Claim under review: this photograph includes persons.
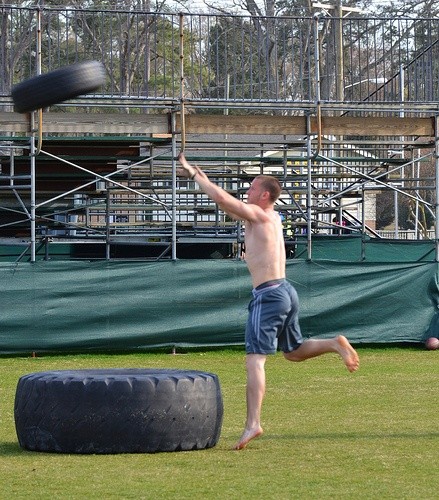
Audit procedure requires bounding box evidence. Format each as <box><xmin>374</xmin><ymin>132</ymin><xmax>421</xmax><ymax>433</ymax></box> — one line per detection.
<box><xmin>179</xmin><ymin>152</ymin><xmax>359</xmax><ymax>449</ymax></box>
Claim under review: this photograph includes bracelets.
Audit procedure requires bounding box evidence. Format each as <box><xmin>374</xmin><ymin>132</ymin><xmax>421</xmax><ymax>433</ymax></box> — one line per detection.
<box><xmin>191</xmin><ymin>171</ymin><xmax>198</xmax><ymax>179</ymax></box>
